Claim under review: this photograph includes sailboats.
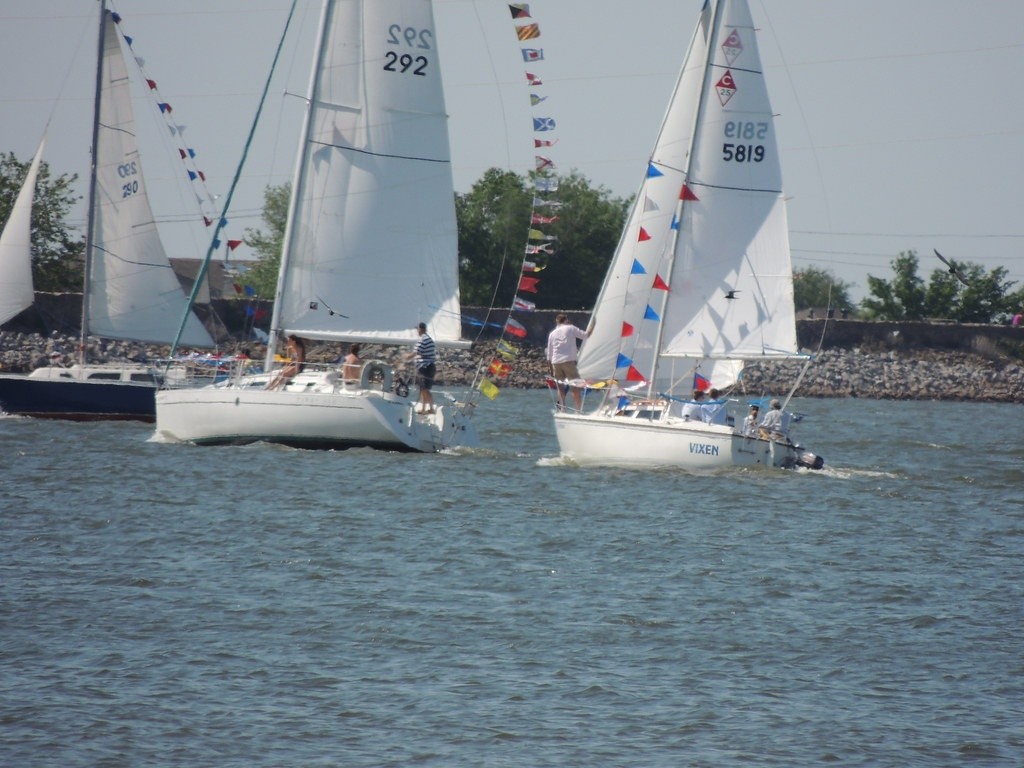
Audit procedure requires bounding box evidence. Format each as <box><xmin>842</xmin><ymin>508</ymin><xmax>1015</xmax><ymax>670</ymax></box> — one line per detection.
<box><xmin>147</xmin><ymin>0</ymin><xmax>557</xmax><ymax>453</ymax></box>
<box><xmin>550</xmin><ymin>0</ymin><xmax>822</xmax><ymax>472</ymax></box>
<box><xmin>1</xmin><ymin>0</ymin><xmax>271</xmax><ymax>419</ymax></box>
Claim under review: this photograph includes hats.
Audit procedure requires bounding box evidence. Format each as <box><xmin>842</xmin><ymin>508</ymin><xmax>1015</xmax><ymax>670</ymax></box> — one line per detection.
<box><xmin>693</xmin><ymin>389</ymin><xmax>704</xmax><ymax>396</ymax></box>
<box><xmin>750</xmin><ymin>404</ymin><xmax>759</xmax><ymax>410</ymax></box>
<box><xmin>769</xmin><ymin>400</ymin><xmax>782</xmax><ymax>408</ymax></box>
<box><xmin>414</xmin><ymin>321</ymin><xmax>427</xmax><ymax>329</ymax></box>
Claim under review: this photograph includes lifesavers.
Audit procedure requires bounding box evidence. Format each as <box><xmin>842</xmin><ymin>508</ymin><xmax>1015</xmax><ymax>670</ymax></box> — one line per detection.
<box><xmin>361</xmin><ymin>359</ymin><xmax>393</xmax><ymax>391</ymax></box>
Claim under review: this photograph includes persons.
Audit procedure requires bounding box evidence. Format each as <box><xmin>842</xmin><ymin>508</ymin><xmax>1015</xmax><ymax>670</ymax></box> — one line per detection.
<box><xmin>701</xmin><ymin>388</ymin><xmax>726</xmax><ymax>426</ymax></box>
<box><xmin>743</xmin><ymin>404</ymin><xmax>765</xmax><ymax>436</ymax></box>
<box><xmin>264</xmin><ymin>335</ymin><xmax>306</xmax><ymax>393</ymax></box>
<box><xmin>682</xmin><ymin>391</ymin><xmax>704</xmax><ymax>421</ymax></box>
<box><xmin>1013</xmin><ymin>312</ymin><xmax>1023</xmax><ymax>328</ymax></box>
<box><xmin>759</xmin><ymin>399</ymin><xmax>797</xmax><ymax>442</ymax></box>
<box><xmin>404</xmin><ymin>322</ymin><xmax>436</xmax><ymax>414</ymax></box>
<box><xmin>547</xmin><ymin>315</ymin><xmax>596</xmax><ymax>414</ymax></box>
<box><xmin>342</xmin><ymin>344</ymin><xmax>364</xmax><ymax>391</ymax></box>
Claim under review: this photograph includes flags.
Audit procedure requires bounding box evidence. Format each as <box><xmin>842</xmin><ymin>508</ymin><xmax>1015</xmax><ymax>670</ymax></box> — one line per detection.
<box><xmin>510</xmin><ymin>4</ymin><xmax>531</xmax><ymax>19</ymax></box>
<box><xmin>516</xmin><ymin>23</ymin><xmax>540</xmax><ymax>40</ymax></box>
<box><xmin>480</xmin><ymin>377</ymin><xmax>500</xmax><ymax>400</ymax></box>
<box><xmin>488</xmin><ymin>358</ymin><xmax>509</xmax><ymax>379</ymax></box>
<box><xmin>522</xmin><ymin>48</ymin><xmax>544</xmax><ymax>62</ymax></box>
<box><xmin>534</xmin><ymin>118</ymin><xmax>555</xmax><ymax>132</ymax></box>
<box><xmin>535</xmin><ymin>139</ymin><xmax>559</xmax><ymax>148</ymax></box>
<box><xmin>527</xmin><ymin>73</ymin><xmax>543</xmax><ymax>86</ymax></box>
<box><xmin>536</xmin><ymin>156</ymin><xmax>557</xmax><ymax>170</ymax></box>
<box><xmin>497</xmin><ymin>177</ymin><xmax>562</xmax><ymax>358</ymax></box>
<box><xmin>531</xmin><ymin>94</ymin><xmax>548</xmax><ymax>106</ymax></box>
